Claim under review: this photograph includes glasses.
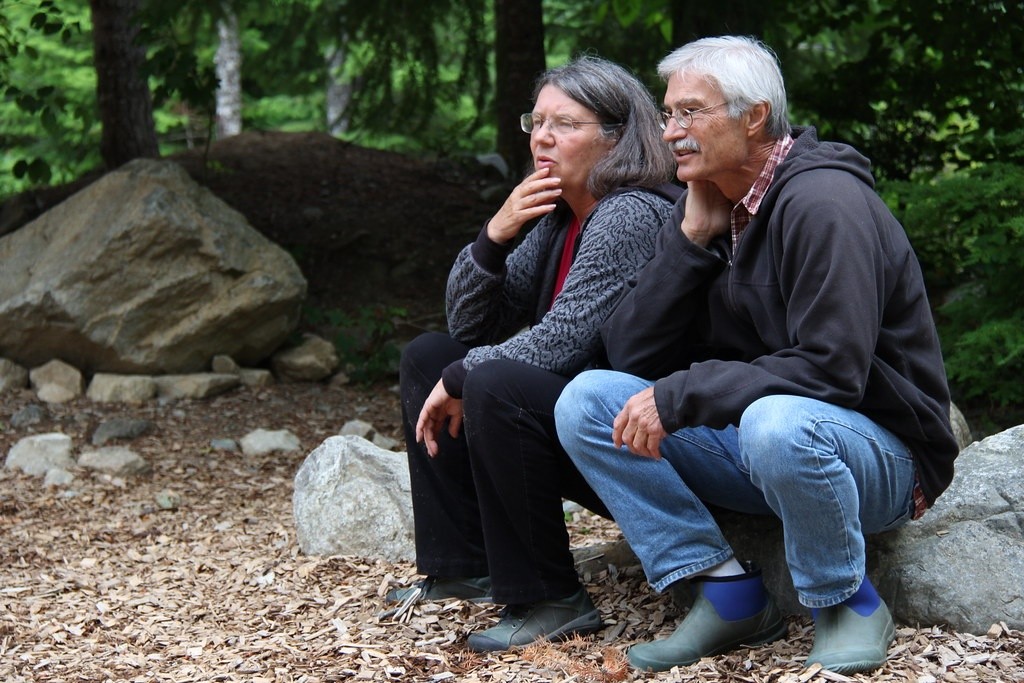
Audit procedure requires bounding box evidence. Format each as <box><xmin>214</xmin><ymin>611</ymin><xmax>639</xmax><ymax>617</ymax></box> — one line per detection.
<box><xmin>521</xmin><ymin>114</ymin><xmax>606</xmax><ymax>134</ymax></box>
<box><xmin>657</xmin><ymin>96</ymin><xmax>742</xmax><ymax>130</ymax></box>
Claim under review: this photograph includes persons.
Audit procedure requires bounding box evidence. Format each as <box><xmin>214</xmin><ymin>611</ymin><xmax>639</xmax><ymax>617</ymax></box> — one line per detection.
<box><xmin>552</xmin><ymin>34</ymin><xmax>960</xmax><ymax>671</ymax></box>
<box><xmin>379</xmin><ymin>57</ymin><xmax>690</xmax><ymax>651</ymax></box>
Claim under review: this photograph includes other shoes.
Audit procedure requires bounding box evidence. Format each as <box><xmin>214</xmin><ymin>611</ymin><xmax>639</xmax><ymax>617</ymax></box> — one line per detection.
<box><xmin>627</xmin><ymin>560</ymin><xmax>788</xmax><ymax>673</ymax></box>
<box><xmin>806</xmin><ymin>573</ymin><xmax>896</xmax><ymax>677</ymax></box>
<box><xmin>468</xmin><ymin>580</ymin><xmax>603</xmax><ymax>654</ymax></box>
<box><xmin>386</xmin><ymin>576</ymin><xmax>493</xmax><ymax>603</ymax></box>
